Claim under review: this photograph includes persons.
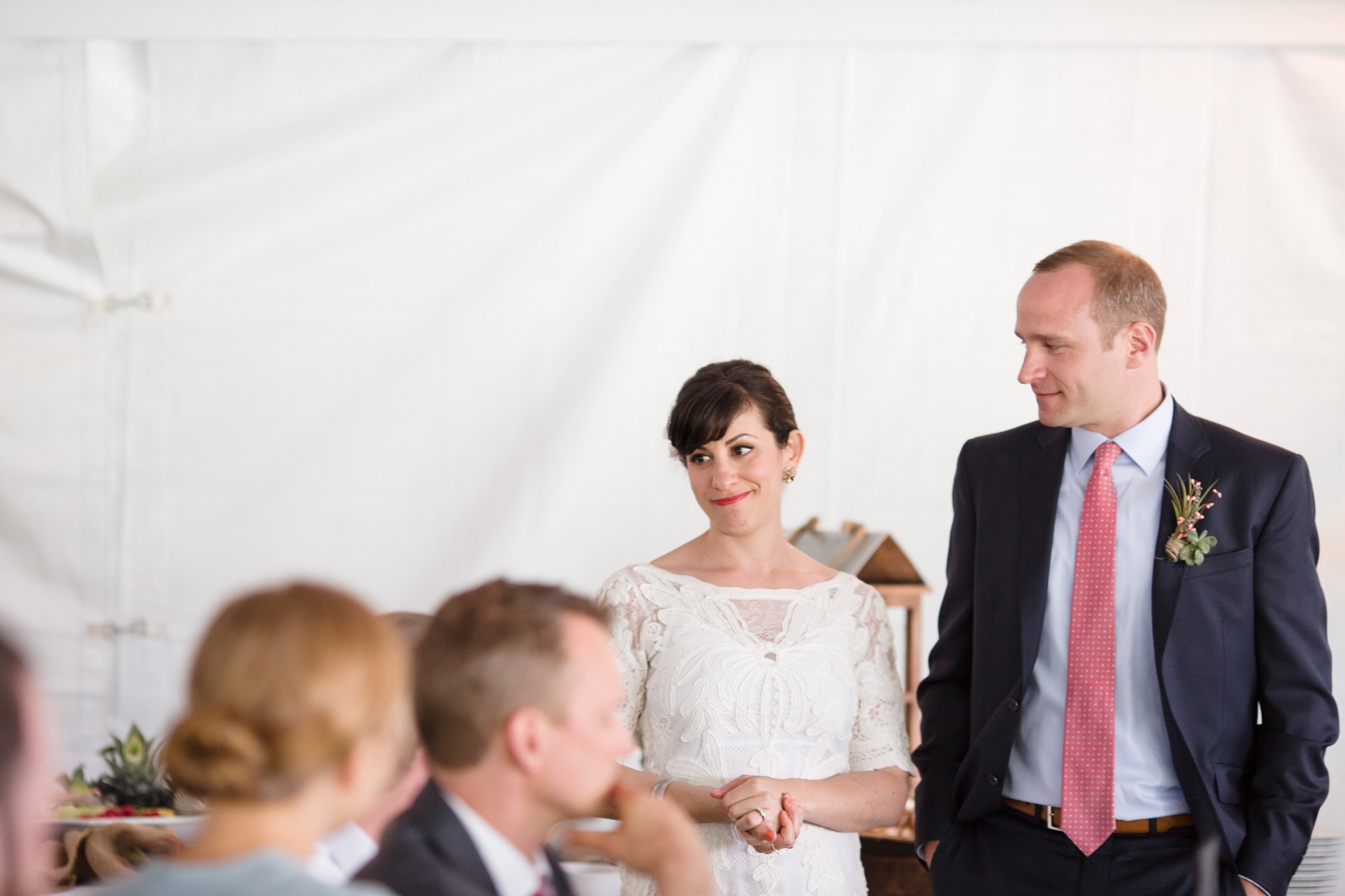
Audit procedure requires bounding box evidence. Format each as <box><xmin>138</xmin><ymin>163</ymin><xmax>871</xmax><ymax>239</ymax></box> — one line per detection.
<box><xmin>314</xmin><ymin>610</ymin><xmax>438</xmax><ymax>882</ymax></box>
<box><xmin>583</xmin><ymin>357</ymin><xmax>914</xmax><ymax>896</ymax></box>
<box><xmin>1</xmin><ymin>636</ymin><xmax>67</xmax><ymax>895</ymax></box>
<box><xmin>80</xmin><ymin>583</ymin><xmax>404</xmax><ymax>895</ymax></box>
<box><xmin>350</xmin><ymin>578</ymin><xmax>722</xmax><ymax>896</ymax></box>
<box><xmin>908</xmin><ymin>238</ymin><xmax>1344</xmax><ymax>896</ymax></box>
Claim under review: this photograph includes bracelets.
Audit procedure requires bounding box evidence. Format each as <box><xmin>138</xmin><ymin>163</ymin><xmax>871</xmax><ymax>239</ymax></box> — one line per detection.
<box><xmin>647</xmin><ymin>778</ymin><xmax>675</xmax><ymax>802</ymax></box>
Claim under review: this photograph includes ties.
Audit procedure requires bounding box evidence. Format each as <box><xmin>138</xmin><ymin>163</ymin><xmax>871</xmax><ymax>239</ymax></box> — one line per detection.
<box><xmin>1059</xmin><ymin>443</ymin><xmax>1127</xmax><ymax>857</ymax></box>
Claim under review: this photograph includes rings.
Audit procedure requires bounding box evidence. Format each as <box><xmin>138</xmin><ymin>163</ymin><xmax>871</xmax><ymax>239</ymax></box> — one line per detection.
<box><xmin>788</xmin><ymin>845</ymin><xmax>794</xmax><ymax>849</ymax></box>
<box><xmin>757</xmin><ymin>807</ymin><xmax>767</xmax><ymax>821</ymax></box>
<box><xmin>771</xmin><ymin>841</ymin><xmax>780</xmax><ymax>854</ymax></box>
<box><xmin>796</xmin><ymin>836</ymin><xmax>799</xmax><ymax>839</ymax></box>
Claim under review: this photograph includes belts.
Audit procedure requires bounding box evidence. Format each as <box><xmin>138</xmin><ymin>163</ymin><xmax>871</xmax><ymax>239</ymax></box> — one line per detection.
<box><xmin>998</xmin><ymin>797</ymin><xmax>1195</xmax><ymax>837</ymax></box>
